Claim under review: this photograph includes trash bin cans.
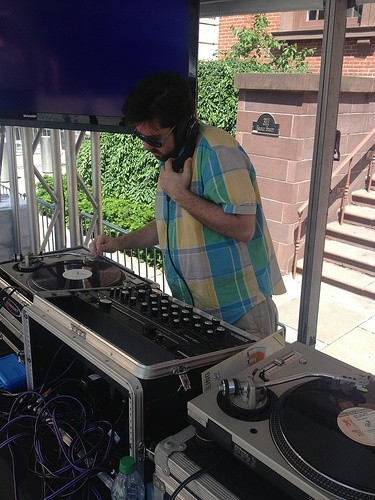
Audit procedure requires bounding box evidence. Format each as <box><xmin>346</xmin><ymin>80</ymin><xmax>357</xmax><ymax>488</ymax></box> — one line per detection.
<box><xmin>1</xmin><ymin>142</ymin><xmax>10</xmax><ymax>183</ymax></box>
<box><xmin>40</xmin><ymin>136</ymin><xmax>54</xmax><ymax>173</ymax></box>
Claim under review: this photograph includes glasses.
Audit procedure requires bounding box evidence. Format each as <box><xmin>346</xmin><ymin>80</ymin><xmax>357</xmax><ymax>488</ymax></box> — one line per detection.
<box><xmin>131</xmin><ymin>126</ymin><xmax>175</xmax><ymax>148</ymax></box>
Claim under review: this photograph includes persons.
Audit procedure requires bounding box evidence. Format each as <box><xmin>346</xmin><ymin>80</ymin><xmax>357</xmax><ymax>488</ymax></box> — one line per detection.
<box><xmin>86</xmin><ymin>68</ymin><xmax>290</xmax><ymax>341</ymax></box>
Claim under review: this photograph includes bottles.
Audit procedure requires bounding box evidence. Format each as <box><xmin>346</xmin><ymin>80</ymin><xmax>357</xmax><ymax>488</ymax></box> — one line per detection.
<box><xmin>111</xmin><ymin>456</ymin><xmax>146</xmax><ymax>500</ymax></box>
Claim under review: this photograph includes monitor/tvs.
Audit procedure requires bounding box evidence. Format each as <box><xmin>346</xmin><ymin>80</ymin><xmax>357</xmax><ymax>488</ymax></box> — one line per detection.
<box><xmin>0</xmin><ymin>0</ymin><xmax>201</xmax><ymax>129</ymax></box>
